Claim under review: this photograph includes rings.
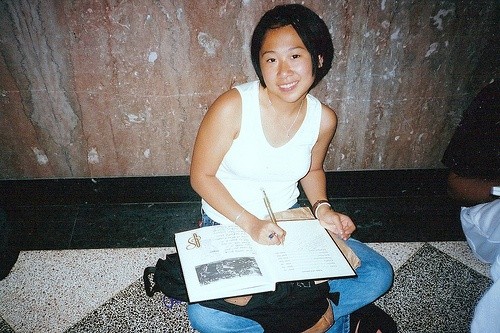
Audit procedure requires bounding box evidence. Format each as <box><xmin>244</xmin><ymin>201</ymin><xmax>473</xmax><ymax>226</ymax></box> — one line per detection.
<box><xmin>268</xmin><ymin>232</ymin><xmax>277</xmax><ymax>240</ymax></box>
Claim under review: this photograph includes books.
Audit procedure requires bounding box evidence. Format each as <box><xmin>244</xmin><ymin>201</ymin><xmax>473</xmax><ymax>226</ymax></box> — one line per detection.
<box><xmin>175</xmin><ymin>219</ymin><xmax>358</xmax><ymax>305</ymax></box>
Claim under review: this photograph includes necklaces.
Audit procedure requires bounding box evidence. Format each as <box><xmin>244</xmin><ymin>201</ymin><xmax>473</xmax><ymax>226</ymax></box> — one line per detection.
<box><xmin>266</xmin><ymin>88</ymin><xmax>303</xmax><ymax>138</ymax></box>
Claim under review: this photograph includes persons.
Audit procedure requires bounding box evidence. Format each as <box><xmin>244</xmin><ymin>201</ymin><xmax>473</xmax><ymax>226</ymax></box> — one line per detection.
<box><xmin>440</xmin><ymin>78</ymin><xmax>500</xmax><ymax>333</ymax></box>
<box><xmin>186</xmin><ymin>4</ymin><xmax>397</xmax><ymax>333</ymax></box>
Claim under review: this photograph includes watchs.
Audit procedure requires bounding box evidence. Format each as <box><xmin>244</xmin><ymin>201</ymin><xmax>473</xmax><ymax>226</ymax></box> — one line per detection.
<box><xmin>489</xmin><ymin>179</ymin><xmax>500</xmax><ymax>200</ymax></box>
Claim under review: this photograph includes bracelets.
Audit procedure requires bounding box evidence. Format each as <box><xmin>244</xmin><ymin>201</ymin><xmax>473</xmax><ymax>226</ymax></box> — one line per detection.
<box><xmin>234</xmin><ymin>209</ymin><xmax>246</xmax><ymax>224</ymax></box>
<box><xmin>311</xmin><ymin>200</ymin><xmax>333</xmax><ymax>219</ymax></box>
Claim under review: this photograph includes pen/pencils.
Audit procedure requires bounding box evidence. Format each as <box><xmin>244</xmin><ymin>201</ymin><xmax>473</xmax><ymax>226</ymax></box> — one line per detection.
<box><xmin>262</xmin><ymin>190</ymin><xmax>284</xmax><ymax>245</ymax></box>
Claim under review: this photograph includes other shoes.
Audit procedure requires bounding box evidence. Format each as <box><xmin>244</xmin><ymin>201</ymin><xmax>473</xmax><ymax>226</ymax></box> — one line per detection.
<box><xmin>350</xmin><ymin>302</ymin><xmax>397</xmax><ymax>333</ymax></box>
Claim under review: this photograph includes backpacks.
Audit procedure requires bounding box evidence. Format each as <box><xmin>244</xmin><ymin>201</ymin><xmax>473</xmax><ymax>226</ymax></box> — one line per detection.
<box><xmin>143</xmin><ymin>253</ymin><xmax>341</xmax><ymax>333</ymax></box>
